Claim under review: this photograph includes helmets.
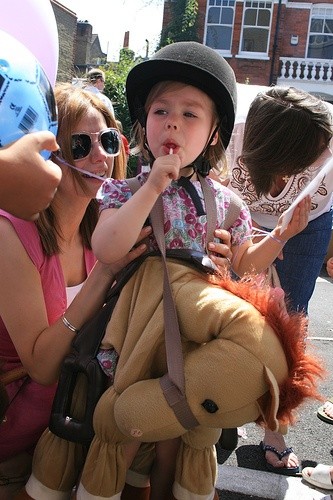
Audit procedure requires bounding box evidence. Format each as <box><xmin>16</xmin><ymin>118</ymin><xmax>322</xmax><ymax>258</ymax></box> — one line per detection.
<box><xmin>125</xmin><ymin>41</ymin><xmax>236</xmax><ymax>172</ymax></box>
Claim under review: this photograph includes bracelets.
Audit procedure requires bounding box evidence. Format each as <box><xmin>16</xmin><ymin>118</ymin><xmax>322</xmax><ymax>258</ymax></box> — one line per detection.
<box><xmin>92</xmin><ymin>42</ymin><xmax>312</xmax><ymax>500</ymax></box>
<box><xmin>61</xmin><ymin>313</ymin><xmax>80</xmax><ymax>334</ymax></box>
<box><xmin>269</xmin><ymin>233</ymin><xmax>287</xmax><ymax>247</ymax></box>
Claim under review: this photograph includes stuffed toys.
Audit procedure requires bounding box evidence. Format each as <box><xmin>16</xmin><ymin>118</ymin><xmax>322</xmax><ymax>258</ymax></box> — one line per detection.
<box><xmin>25</xmin><ymin>254</ymin><xmax>328</xmax><ymax>500</ymax></box>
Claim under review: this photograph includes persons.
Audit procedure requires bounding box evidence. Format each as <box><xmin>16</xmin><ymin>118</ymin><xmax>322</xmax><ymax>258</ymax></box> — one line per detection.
<box><xmin>302</xmin><ymin>256</ymin><xmax>333</xmax><ymax>491</ymax></box>
<box><xmin>0</xmin><ymin>130</ymin><xmax>63</xmax><ymax>220</ymax></box>
<box><xmin>209</xmin><ymin>87</ymin><xmax>333</xmax><ymax>475</ymax></box>
<box><xmin>0</xmin><ymin>83</ymin><xmax>231</xmax><ymax>500</ymax></box>
<box><xmin>83</xmin><ymin>69</ymin><xmax>115</xmax><ymax>120</ymax></box>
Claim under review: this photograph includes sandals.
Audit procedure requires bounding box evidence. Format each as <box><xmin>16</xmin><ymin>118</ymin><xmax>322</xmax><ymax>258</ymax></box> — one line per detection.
<box><xmin>317</xmin><ymin>397</ymin><xmax>333</xmax><ymax>425</ymax></box>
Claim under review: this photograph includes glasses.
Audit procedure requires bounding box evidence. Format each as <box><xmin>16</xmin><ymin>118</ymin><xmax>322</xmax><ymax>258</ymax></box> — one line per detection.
<box><xmin>68</xmin><ymin>127</ymin><xmax>122</xmax><ymax>161</ymax></box>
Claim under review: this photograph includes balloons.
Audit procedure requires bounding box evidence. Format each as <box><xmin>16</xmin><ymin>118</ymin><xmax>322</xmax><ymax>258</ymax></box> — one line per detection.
<box><xmin>0</xmin><ymin>0</ymin><xmax>60</xmax><ymax>88</ymax></box>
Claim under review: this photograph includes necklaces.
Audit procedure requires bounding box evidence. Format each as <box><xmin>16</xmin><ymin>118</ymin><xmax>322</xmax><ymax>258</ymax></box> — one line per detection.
<box><xmin>282</xmin><ymin>175</ymin><xmax>290</xmax><ymax>184</ymax></box>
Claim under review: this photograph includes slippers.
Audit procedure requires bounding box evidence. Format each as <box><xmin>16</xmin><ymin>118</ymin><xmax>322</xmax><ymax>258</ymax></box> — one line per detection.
<box><xmin>260</xmin><ymin>441</ymin><xmax>300</xmax><ymax>473</ymax></box>
<box><xmin>302</xmin><ymin>464</ymin><xmax>333</xmax><ymax>489</ymax></box>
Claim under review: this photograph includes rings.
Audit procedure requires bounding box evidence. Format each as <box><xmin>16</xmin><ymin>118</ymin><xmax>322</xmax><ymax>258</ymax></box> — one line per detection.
<box><xmin>226</xmin><ymin>257</ymin><xmax>230</xmax><ymax>264</ymax></box>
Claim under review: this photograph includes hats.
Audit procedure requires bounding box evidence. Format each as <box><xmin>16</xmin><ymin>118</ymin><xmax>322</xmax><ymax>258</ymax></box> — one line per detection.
<box><xmin>87</xmin><ymin>69</ymin><xmax>106</xmax><ymax>80</ymax></box>
